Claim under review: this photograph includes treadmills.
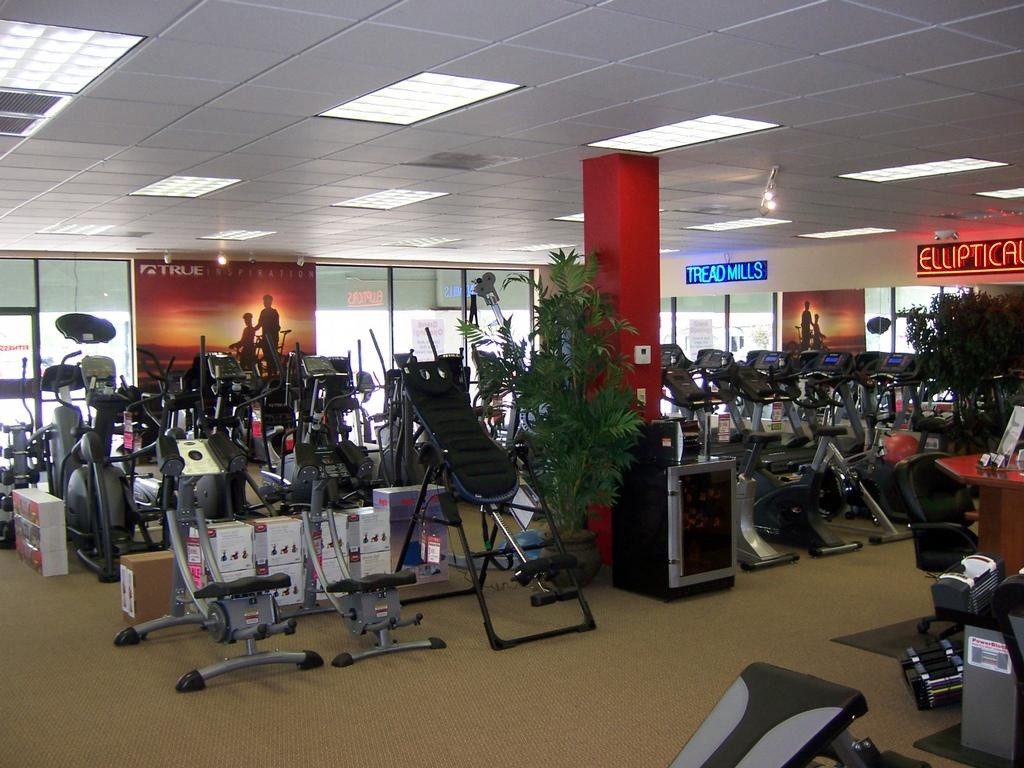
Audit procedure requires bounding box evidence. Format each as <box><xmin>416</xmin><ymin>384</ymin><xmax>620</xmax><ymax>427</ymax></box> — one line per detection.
<box><xmin>660</xmin><ymin>343</ymin><xmax>926</xmax><ymax>474</ymax></box>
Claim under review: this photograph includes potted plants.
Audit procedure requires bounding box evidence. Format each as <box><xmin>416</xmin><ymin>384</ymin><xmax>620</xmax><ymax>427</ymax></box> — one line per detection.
<box><xmin>455</xmin><ymin>245</ymin><xmax>649</xmax><ymax>588</ymax></box>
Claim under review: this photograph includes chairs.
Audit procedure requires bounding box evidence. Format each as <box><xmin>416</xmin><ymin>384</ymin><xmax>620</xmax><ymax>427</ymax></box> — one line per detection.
<box><xmin>892</xmin><ymin>450</ymin><xmax>981</xmax><ymax>573</ymax></box>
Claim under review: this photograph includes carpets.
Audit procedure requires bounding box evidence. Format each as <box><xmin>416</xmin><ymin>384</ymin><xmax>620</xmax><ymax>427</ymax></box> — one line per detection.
<box><xmin>913</xmin><ymin>723</ymin><xmax>1024</xmax><ymax>768</ymax></box>
<box><xmin>829</xmin><ymin>614</ymin><xmax>964</xmax><ymax>659</ymax></box>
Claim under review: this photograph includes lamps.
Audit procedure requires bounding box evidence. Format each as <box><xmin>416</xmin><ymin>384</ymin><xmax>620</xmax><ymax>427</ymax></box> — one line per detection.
<box><xmin>934</xmin><ymin>231</ymin><xmax>960</xmax><ymax>241</ymax></box>
<box><xmin>760</xmin><ymin>166</ymin><xmax>781</xmax><ymax>215</ymax></box>
<box><xmin>248</xmin><ymin>252</ymin><xmax>256</xmax><ymax>264</ymax></box>
<box><xmin>164</xmin><ymin>249</ymin><xmax>172</xmax><ymax>264</ymax></box>
<box><xmin>296</xmin><ymin>255</ymin><xmax>305</xmax><ymax>266</ymax></box>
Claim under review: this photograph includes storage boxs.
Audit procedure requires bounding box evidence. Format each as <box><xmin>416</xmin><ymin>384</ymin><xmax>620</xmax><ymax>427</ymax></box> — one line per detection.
<box><xmin>120</xmin><ymin>551</ymin><xmax>176</xmax><ymax>626</ymax></box>
<box><xmin>187</xmin><ymin>484</ymin><xmax>450</xmax><ymax>613</ymax></box>
<box><xmin>12</xmin><ymin>488</ymin><xmax>68</xmax><ymax>576</ymax></box>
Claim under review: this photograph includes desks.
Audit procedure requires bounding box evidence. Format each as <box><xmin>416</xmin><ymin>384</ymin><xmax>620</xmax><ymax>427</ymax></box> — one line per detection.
<box><xmin>935</xmin><ymin>453</ymin><xmax>1024</xmax><ymax>579</ymax></box>
